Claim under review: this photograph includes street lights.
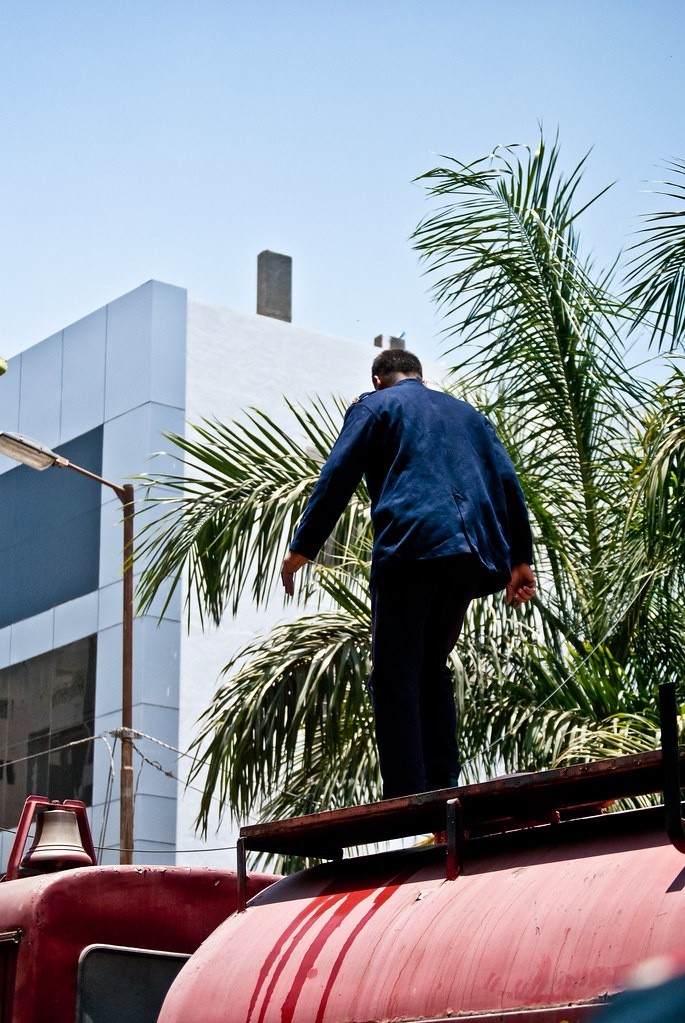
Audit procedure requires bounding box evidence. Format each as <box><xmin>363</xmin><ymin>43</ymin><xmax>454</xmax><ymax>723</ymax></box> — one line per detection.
<box><xmin>0</xmin><ymin>430</ymin><xmax>134</xmax><ymax>865</ymax></box>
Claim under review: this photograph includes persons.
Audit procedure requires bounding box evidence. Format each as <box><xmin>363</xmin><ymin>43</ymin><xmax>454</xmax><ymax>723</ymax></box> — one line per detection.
<box><xmin>278</xmin><ymin>349</ymin><xmax>538</xmax><ymax>803</ymax></box>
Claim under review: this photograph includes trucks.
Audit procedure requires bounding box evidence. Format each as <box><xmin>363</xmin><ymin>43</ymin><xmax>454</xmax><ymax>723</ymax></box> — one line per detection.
<box><xmin>0</xmin><ymin>683</ymin><xmax>685</xmax><ymax>1023</ymax></box>
